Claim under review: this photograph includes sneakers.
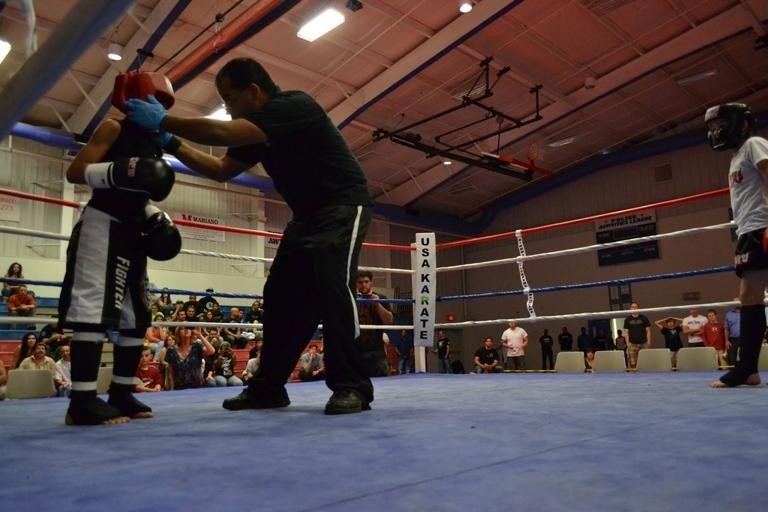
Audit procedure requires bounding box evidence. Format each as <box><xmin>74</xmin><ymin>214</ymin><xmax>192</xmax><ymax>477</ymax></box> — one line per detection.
<box><xmin>222</xmin><ymin>383</ymin><xmax>290</xmax><ymax>410</ymax></box>
<box><xmin>325</xmin><ymin>390</ymin><xmax>363</xmax><ymax>413</ymax></box>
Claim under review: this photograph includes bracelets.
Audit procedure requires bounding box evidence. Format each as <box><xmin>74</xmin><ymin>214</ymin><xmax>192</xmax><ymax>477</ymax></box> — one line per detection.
<box><xmin>162</xmin><ymin>136</ymin><xmax>180</xmax><ymax>155</ymax></box>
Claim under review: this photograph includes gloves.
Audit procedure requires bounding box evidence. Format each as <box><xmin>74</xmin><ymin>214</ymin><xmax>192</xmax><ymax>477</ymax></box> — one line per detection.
<box><xmin>126</xmin><ymin>94</ymin><xmax>173</xmax><ymax>147</ymax></box>
<box><xmin>113</xmin><ymin>158</ymin><xmax>174</xmax><ymax>201</ymax></box>
<box><xmin>144</xmin><ymin>211</ymin><xmax>181</xmax><ymax>260</ymax></box>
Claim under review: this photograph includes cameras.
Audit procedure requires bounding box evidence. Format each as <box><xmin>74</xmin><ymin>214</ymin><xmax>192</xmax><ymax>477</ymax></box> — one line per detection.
<box><xmin>186</xmin><ymin>326</ymin><xmax>195</xmax><ymax>330</ymax></box>
<box><xmin>357</xmin><ymin>294</ymin><xmax>372</xmax><ymax>300</ymax></box>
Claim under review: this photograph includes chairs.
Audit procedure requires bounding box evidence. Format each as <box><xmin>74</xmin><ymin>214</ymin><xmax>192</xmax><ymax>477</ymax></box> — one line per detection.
<box><xmin>552</xmin><ymin>342</ymin><xmax>767</xmax><ymax>378</ymax></box>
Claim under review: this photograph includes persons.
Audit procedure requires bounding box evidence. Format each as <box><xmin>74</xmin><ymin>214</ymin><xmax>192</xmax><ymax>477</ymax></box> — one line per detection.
<box><xmin>431</xmin><ymin>329</ymin><xmax>450</xmax><ymax>375</ymax></box>
<box><xmin>57</xmin><ymin>70</ymin><xmax>181</xmax><ymax>426</ymax></box>
<box><xmin>473</xmin><ymin>289</ymin><xmax>767</xmax><ymax>374</ymax></box>
<box><xmin>706</xmin><ymin>103</ymin><xmax>768</xmax><ymax>387</ymax></box>
<box><xmin>122</xmin><ymin>57</ymin><xmax>377</xmax><ymax>415</ymax></box>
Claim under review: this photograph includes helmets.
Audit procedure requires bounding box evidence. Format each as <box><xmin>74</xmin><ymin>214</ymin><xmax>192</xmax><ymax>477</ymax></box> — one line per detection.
<box><xmin>111</xmin><ymin>72</ymin><xmax>175</xmax><ymax>114</ymax></box>
<box><xmin>705</xmin><ymin>104</ymin><xmax>754</xmax><ymax>152</ymax></box>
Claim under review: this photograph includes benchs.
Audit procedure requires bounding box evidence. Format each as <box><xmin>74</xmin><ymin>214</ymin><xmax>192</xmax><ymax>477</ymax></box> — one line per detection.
<box><xmin>0</xmin><ymin>295</ymin><xmax>324</xmax><ymax>403</ymax></box>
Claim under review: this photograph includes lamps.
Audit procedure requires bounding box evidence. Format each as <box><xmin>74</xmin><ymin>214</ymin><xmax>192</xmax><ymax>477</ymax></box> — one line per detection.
<box><xmin>105</xmin><ymin>43</ymin><xmax>124</xmax><ymax>61</ymax></box>
